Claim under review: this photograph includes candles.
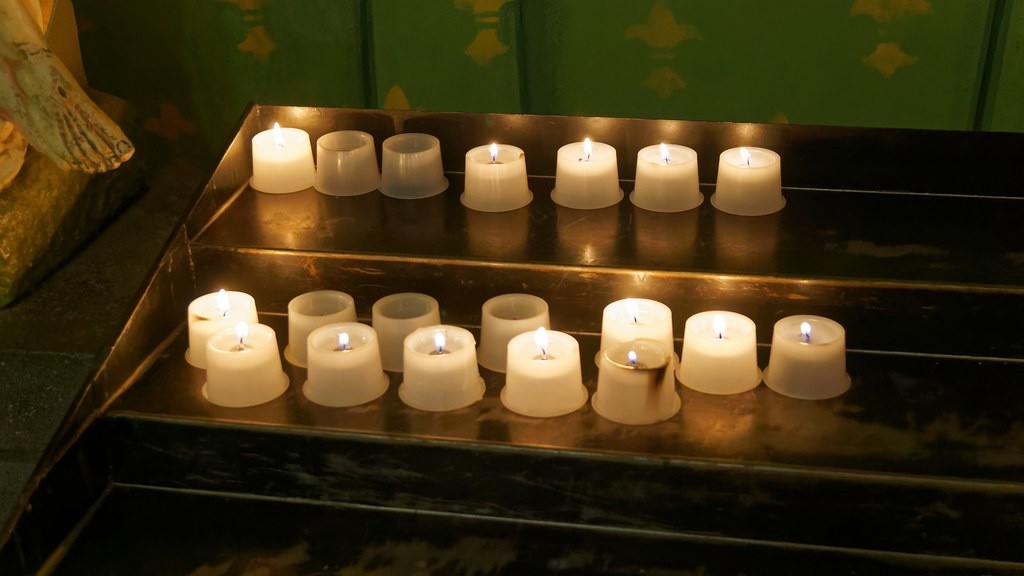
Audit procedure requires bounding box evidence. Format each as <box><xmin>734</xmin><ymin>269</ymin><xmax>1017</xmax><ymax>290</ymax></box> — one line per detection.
<box><xmin>372</xmin><ymin>293</ymin><xmax>440</xmax><ymax>373</ymax></box>
<box><xmin>710</xmin><ymin>146</ymin><xmax>787</xmax><ymax>216</ymax></box>
<box><xmin>762</xmin><ymin>313</ymin><xmax>852</xmax><ymax>401</ymax></box>
<box><xmin>398</xmin><ymin>324</ymin><xmax>486</xmax><ymax>412</ymax></box>
<box><xmin>591</xmin><ymin>336</ymin><xmax>681</xmax><ymax>425</ymax></box>
<box><xmin>283</xmin><ymin>290</ymin><xmax>356</xmax><ymax>368</ymax></box>
<box><xmin>201</xmin><ymin>319</ymin><xmax>290</xmax><ymax>408</ymax></box>
<box><xmin>594</xmin><ymin>297</ymin><xmax>679</xmax><ymax>372</ymax></box>
<box><xmin>500</xmin><ymin>328</ymin><xmax>589</xmax><ymax>418</ymax></box>
<box><xmin>459</xmin><ymin>141</ymin><xmax>534</xmax><ymax>213</ymax></box>
<box><xmin>185</xmin><ymin>288</ymin><xmax>259</xmax><ymax>370</ymax></box>
<box><xmin>675</xmin><ymin>311</ymin><xmax>763</xmax><ymax>395</ymax></box>
<box><xmin>313</xmin><ymin>130</ymin><xmax>381</xmax><ymax>196</ymax></box>
<box><xmin>550</xmin><ymin>137</ymin><xmax>624</xmax><ymax>210</ymax></box>
<box><xmin>302</xmin><ymin>321</ymin><xmax>391</xmax><ymax>407</ymax></box>
<box><xmin>249</xmin><ymin>120</ymin><xmax>315</xmax><ymax>194</ymax></box>
<box><xmin>377</xmin><ymin>133</ymin><xmax>449</xmax><ymax>199</ymax></box>
<box><xmin>629</xmin><ymin>141</ymin><xmax>704</xmax><ymax>212</ymax></box>
<box><xmin>476</xmin><ymin>293</ymin><xmax>551</xmax><ymax>373</ymax></box>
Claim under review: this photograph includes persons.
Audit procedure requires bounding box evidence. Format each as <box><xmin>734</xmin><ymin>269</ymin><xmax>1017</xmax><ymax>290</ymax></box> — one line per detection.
<box><xmin>2</xmin><ymin>1</ymin><xmax>135</xmax><ymax>174</ymax></box>
